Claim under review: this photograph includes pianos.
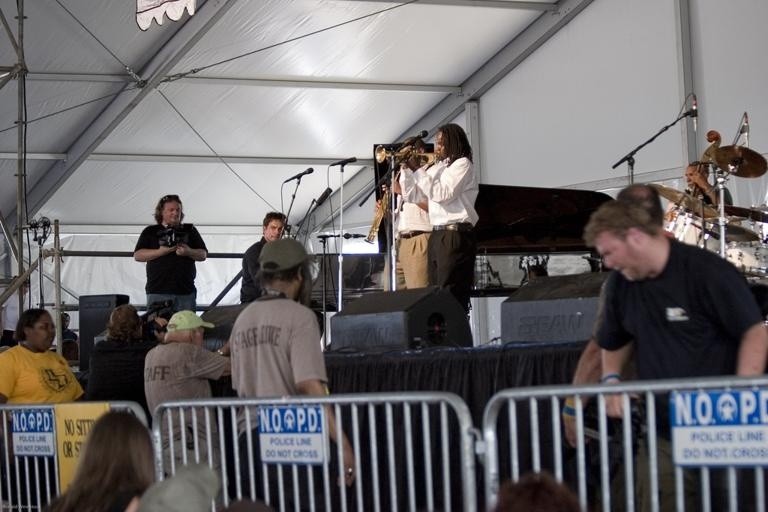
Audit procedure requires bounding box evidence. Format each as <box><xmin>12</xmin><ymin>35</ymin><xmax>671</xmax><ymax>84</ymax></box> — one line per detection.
<box><xmin>475</xmin><ymin>183</ymin><xmax>618</xmax><ymax>274</ymax></box>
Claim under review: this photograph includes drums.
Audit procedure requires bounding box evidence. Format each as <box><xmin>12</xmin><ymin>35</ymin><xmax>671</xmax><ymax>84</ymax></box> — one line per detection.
<box><xmin>720</xmin><ymin>240</ymin><xmax>767</xmax><ymax>284</ymax></box>
<box><xmin>669</xmin><ymin>213</ymin><xmax>720</xmax><ymax>255</ymax></box>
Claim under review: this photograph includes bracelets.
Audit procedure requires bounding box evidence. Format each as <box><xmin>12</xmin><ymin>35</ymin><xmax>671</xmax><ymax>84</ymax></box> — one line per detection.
<box><xmin>703</xmin><ymin>183</ymin><xmax>712</xmax><ymax>192</ymax></box>
<box><xmin>218</xmin><ymin>349</ymin><xmax>224</xmax><ymax>355</ymax></box>
<box><xmin>601</xmin><ymin>372</ymin><xmax>621</xmax><ymax>384</ymax></box>
<box><xmin>563</xmin><ymin>395</ymin><xmax>576</xmax><ymax>420</ymax></box>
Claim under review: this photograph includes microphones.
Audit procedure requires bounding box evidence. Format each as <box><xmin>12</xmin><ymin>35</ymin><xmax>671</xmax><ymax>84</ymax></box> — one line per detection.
<box><xmin>691</xmin><ymin>98</ymin><xmax>699</xmax><ymax>134</ymax></box>
<box><xmin>314</xmin><ymin>186</ymin><xmax>333</xmax><ymax>206</ymax></box>
<box><xmin>744</xmin><ymin>114</ymin><xmax>750</xmax><ymax>148</ymax></box>
<box><xmin>284</xmin><ymin>166</ymin><xmax>314</xmax><ymax>181</ymax></box>
<box><xmin>405</xmin><ymin>129</ymin><xmax>428</xmax><ymax>146</ymax></box>
<box><xmin>331</xmin><ymin>155</ymin><xmax>358</xmax><ymax>168</ymax></box>
<box><xmin>352</xmin><ymin>232</ymin><xmax>367</xmax><ymax>239</ymax></box>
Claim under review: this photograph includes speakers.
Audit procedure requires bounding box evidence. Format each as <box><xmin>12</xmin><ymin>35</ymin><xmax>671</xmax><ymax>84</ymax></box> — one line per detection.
<box><xmin>331</xmin><ymin>286</ymin><xmax>474</xmax><ymax>351</ymax></box>
<box><xmin>199</xmin><ymin>304</ymin><xmax>249</xmax><ymax>340</ymax></box>
<box><xmin>500</xmin><ymin>270</ymin><xmax>614</xmax><ymax>343</ymax></box>
<box><xmin>79</xmin><ymin>294</ymin><xmax>131</xmax><ymax>370</ymax></box>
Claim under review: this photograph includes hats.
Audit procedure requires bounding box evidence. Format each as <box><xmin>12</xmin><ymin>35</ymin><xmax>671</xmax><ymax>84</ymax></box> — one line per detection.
<box><xmin>259</xmin><ymin>238</ymin><xmax>309</xmax><ymax>273</ymax></box>
<box><xmin>165</xmin><ymin>309</ymin><xmax>215</xmax><ymax>333</ymax></box>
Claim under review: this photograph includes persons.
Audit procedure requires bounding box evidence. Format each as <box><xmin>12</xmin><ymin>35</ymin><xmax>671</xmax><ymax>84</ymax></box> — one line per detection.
<box><xmin>134</xmin><ymin>195</ymin><xmax>208</xmax><ymax>313</ymax></box>
<box><xmin>0</xmin><ymin>278</ymin><xmax>36</xmax><ymax>347</ymax></box>
<box><xmin>0</xmin><ymin>308</ymin><xmax>84</xmax><ymax>512</ymax></box>
<box><xmin>584</xmin><ymin>200</ymin><xmax>768</xmax><ymax>511</ymax></box>
<box><xmin>374</xmin><ymin>136</ymin><xmax>433</xmax><ymax>293</ymax></box>
<box><xmin>561</xmin><ymin>182</ymin><xmax>674</xmax><ymax>512</ymax></box>
<box><xmin>61</xmin><ymin>313</ymin><xmax>77</xmax><ymax>341</ymax></box>
<box><xmin>240</xmin><ymin>212</ymin><xmax>287</xmax><ymax>303</ymax></box>
<box><xmin>666</xmin><ymin>160</ymin><xmax>734</xmax><ymax>219</ymax></box>
<box><xmin>229</xmin><ymin>238</ymin><xmax>359</xmax><ymax>511</ymax></box>
<box><xmin>145</xmin><ymin>310</ymin><xmax>231</xmax><ymax>512</ymax></box>
<box><xmin>493</xmin><ymin>470</ymin><xmax>579</xmax><ymax>510</ymax></box>
<box><xmin>399</xmin><ymin>125</ymin><xmax>479</xmax><ymax>312</ymax></box>
<box><xmin>86</xmin><ymin>305</ymin><xmax>167</xmax><ymax>429</ymax></box>
<box><xmin>41</xmin><ymin>410</ymin><xmax>156</xmax><ymax>512</ymax></box>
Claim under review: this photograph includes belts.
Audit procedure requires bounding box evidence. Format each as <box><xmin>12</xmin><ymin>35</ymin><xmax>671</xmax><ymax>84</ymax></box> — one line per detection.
<box><xmin>399</xmin><ymin>230</ymin><xmax>423</xmax><ymax>239</ymax></box>
<box><xmin>433</xmin><ymin>224</ymin><xmax>470</xmax><ymax>233</ymax></box>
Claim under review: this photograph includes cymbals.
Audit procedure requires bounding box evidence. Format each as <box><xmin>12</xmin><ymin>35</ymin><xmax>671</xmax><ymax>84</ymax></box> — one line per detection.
<box><xmin>711</xmin><ymin>147</ymin><xmax>766</xmax><ymax>177</ymax></box>
<box><xmin>706</xmin><ymin>224</ymin><xmax>760</xmax><ymax>243</ymax></box>
<box><xmin>650</xmin><ymin>183</ymin><xmax>720</xmax><ymax>216</ymax></box>
<box><xmin>709</xmin><ymin>206</ymin><xmax>767</xmax><ymax>222</ymax></box>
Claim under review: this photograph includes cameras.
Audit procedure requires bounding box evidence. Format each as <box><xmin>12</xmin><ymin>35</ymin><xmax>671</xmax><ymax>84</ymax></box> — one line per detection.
<box><xmin>161</xmin><ymin>223</ymin><xmax>186</xmax><ymax>246</ymax></box>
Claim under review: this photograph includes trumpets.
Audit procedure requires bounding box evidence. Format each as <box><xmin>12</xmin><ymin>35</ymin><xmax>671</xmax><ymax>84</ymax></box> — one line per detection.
<box><xmin>375</xmin><ymin>145</ymin><xmax>435</xmax><ymax>162</ymax></box>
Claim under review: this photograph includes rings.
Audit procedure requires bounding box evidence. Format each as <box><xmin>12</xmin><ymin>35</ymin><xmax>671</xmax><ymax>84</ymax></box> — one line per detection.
<box><xmin>349</xmin><ymin>468</ymin><xmax>353</xmax><ymax>476</ymax></box>
